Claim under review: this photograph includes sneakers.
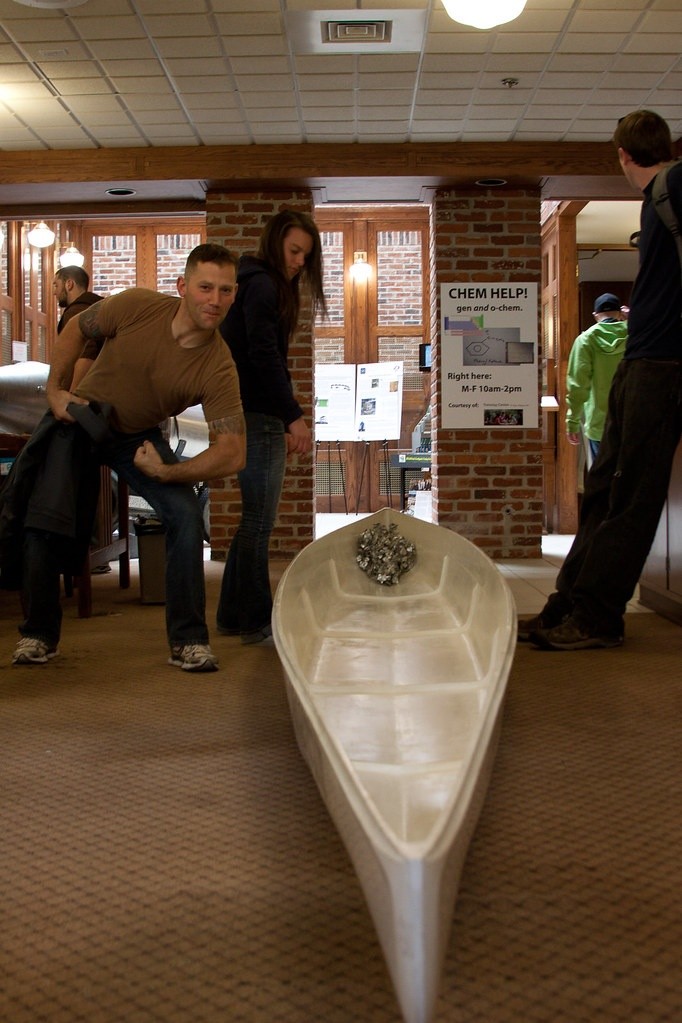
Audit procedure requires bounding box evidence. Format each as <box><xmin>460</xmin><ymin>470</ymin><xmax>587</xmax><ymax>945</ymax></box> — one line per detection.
<box><xmin>12</xmin><ymin>636</ymin><xmax>59</xmax><ymax>664</ymax></box>
<box><xmin>540</xmin><ymin>614</ymin><xmax>624</xmax><ymax>650</ymax></box>
<box><xmin>517</xmin><ymin>594</ymin><xmax>574</xmax><ymax>640</ymax></box>
<box><xmin>168</xmin><ymin>639</ymin><xmax>220</xmax><ymax>672</ymax></box>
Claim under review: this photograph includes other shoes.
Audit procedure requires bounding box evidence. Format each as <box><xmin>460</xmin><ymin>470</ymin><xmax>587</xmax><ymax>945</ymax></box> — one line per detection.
<box><xmin>91</xmin><ymin>561</ymin><xmax>111</xmax><ymax>573</ymax></box>
<box><xmin>245</xmin><ymin>635</ymin><xmax>275</xmax><ymax>647</ymax></box>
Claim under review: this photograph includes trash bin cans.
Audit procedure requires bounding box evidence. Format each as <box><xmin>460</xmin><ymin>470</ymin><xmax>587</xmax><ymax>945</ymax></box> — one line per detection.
<box><xmin>133</xmin><ymin>514</ymin><xmax>166</xmax><ymax>604</ymax></box>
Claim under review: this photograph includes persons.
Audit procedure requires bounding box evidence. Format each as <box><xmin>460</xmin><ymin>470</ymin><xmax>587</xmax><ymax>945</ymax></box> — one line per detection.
<box><xmin>484</xmin><ymin>410</ymin><xmax>517</xmax><ymax>424</ymax></box>
<box><xmin>359</xmin><ymin>422</ymin><xmax>365</xmax><ymax>432</ymax></box>
<box><xmin>412</xmin><ymin>479</ymin><xmax>431</xmax><ymax>490</ymax></box>
<box><xmin>12</xmin><ymin>242</ymin><xmax>247</xmax><ymax>674</ymax></box>
<box><xmin>566</xmin><ymin>292</ymin><xmax>630</xmax><ymax>457</ymax></box>
<box><xmin>518</xmin><ymin>106</ymin><xmax>682</xmax><ymax>647</ymax></box>
<box><xmin>53</xmin><ymin>267</ymin><xmax>111</xmax><ymax>573</ymax></box>
<box><xmin>215</xmin><ymin>210</ymin><xmax>328</xmax><ymax>644</ymax></box>
<box><xmin>316</xmin><ymin>416</ymin><xmax>327</xmax><ymax>424</ymax></box>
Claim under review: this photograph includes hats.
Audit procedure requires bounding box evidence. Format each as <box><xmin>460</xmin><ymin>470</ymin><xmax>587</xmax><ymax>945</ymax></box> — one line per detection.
<box><xmin>594</xmin><ymin>292</ymin><xmax>620</xmax><ymax>313</ymax></box>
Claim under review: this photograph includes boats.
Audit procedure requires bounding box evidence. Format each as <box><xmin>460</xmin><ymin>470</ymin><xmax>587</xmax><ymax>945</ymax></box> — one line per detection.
<box><xmin>269</xmin><ymin>505</ymin><xmax>518</xmax><ymax>1022</ymax></box>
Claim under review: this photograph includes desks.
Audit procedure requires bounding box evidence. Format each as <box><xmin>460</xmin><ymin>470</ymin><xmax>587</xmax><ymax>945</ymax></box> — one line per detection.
<box><xmin>0</xmin><ymin>433</ymin><xmax>131</xmax><ymax>622</ymax></box>
<box><xmin>390</xmin><ymin>453</ymin><xmax>432</xmax><ymax>513</ymax></box>
<box><xmin>540</xmin><ymin>395</ymin><xmax>560</xmax><ymax>411</ymax></box>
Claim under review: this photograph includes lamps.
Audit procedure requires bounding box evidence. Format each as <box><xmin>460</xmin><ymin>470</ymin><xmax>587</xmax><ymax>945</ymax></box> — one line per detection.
<box><xmin>441</xmin><ymin>0</ymin><xmax>528</xmax><ymax>30</ymax></box>
<box><xmin>26</xmin><ymin>220</ymin><xmax>56</xmax><ymax>249</ymax></box>
<box><xmin>60</xmin><ymin>242</ymin><xmax>85</xmax><ymax>268</ymax></box>
